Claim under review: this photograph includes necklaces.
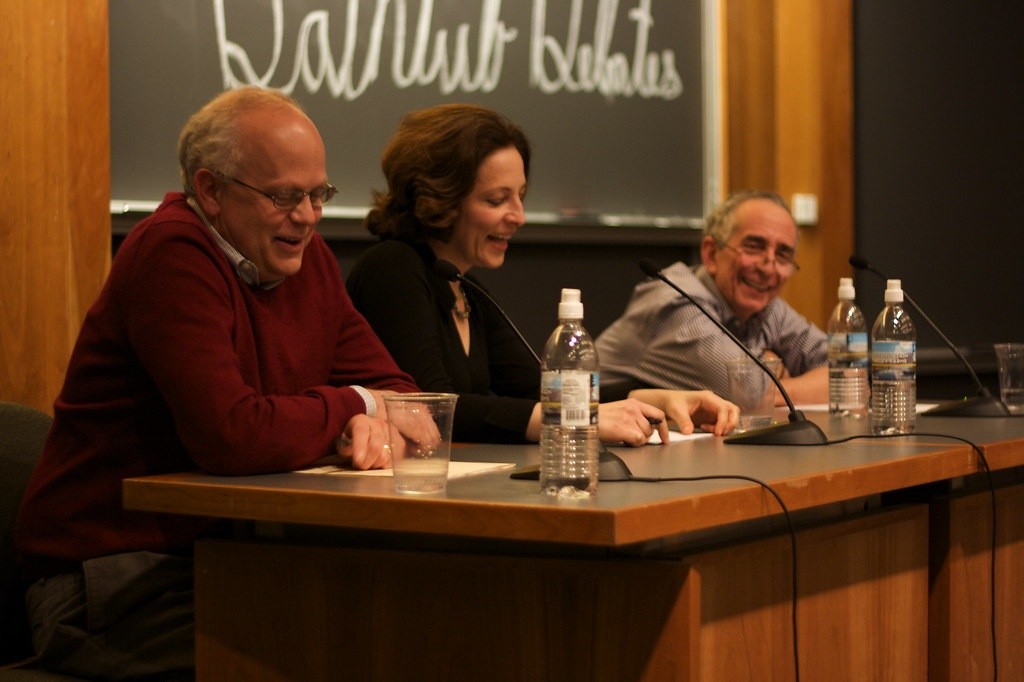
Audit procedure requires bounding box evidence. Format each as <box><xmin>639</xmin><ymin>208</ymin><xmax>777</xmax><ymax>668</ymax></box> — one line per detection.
<box><xmin>453</xmin><ymin>287</ymin><xmax>470</xmax><ymax>319</ymax></box>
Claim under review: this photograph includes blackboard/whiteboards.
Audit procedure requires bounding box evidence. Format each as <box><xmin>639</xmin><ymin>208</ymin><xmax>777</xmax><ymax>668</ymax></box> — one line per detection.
<box><xmin>109</xmin><ymin>2</ymin><xmax>726</xmax><ymax>230</ymax></box>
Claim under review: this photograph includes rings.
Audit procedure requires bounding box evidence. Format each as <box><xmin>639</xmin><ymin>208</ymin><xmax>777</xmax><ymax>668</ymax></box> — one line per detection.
<box><xmin>384</xmin><ymin>445</ymin><xmax>391</xmax><ymax>454</ymax></box>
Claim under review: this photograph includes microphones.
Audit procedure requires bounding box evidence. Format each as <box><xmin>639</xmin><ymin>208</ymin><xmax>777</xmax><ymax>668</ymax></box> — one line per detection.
<box><xmin>847</xmin><ymin>254</ymin><xmax>1011</xmax><ymax>418</ymax></box>
<box><xmin>639</xmin><ymin>256</ymin><xmax>829</xmax><ymax>445</ymax></box>
<box><xmin>430</xmin><ymin>258</ymin><xmax>633</xmax><ymax>482</ymax></box>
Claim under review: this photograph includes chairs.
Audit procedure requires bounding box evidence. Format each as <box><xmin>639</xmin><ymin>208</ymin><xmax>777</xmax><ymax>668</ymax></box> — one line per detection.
<box><xmin>0</xmin><ymin>402</ymin><xmax>107</xmax><ymax>682</ymax></box>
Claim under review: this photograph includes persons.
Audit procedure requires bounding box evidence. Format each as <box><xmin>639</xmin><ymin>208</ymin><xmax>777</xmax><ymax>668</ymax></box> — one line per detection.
<box><xmin>583</xmin><ymin>188</ymin><xmax>863</xmax><ymax>415</ymax></box>
<box><xmin>15</xmin><ymin>85</ymin><xmax>441</xmax><ymax>682</ymax></box>
<box><xmin>345</xmin><ymin>104</ymin><xmax>740</xmax><ymax>446</ymax></box>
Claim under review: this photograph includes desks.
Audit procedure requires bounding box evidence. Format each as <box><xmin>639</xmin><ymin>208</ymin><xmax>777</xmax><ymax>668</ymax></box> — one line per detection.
<box><xmin>123</xmin><ymin>415</ymin><xmax>1024</xmax><ymax>681</ymax></box>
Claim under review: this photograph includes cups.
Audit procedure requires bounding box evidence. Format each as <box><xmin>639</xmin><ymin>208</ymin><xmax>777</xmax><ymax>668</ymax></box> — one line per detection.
<box><xmin>995</xmin><ymin>343</ymin><xmax>1024</xmax><ymax>413</ymax></box>
<box><xmin>726</xmin><ymin>357</ymin><xmax>784</xmax><ymax>431</ymax></box>
<box><xmin>384</xmin><ymin>393</ymin><xmax>458</xmax><ymax>494</ymax></box>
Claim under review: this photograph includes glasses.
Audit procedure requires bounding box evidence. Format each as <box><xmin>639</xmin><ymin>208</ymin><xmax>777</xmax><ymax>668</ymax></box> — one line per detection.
<box><xmin>208</xmin><ymin>167</ymin><xmax>338</xmax><ymax>210</ymax></box>
<box><xmin>714</xmin><ymin>237</ymin><xmax>801</xmax><ymax>277</ymax></box>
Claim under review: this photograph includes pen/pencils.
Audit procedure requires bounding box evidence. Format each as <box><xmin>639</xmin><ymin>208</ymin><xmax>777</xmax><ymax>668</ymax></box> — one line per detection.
<box><xmin>648</xmin><ymin>418</ymin><xmax>663</xmax><ymax>425</ymax></box>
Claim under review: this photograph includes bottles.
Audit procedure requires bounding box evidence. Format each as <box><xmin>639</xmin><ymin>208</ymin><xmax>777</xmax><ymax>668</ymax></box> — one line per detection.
<box><xmin>871</xmin><ymin>279</ymin><xmax>920</xmax><ymax>437</ymax></box>
<box><xmin>540</xmin><ymin>288</ymin><xmax>599</xmax><ymax>500</ymax></box>
<box><xmin>828</xmin><ymin>277</ymin><xmax>869</xmax><ymax>421</ymax></box>
